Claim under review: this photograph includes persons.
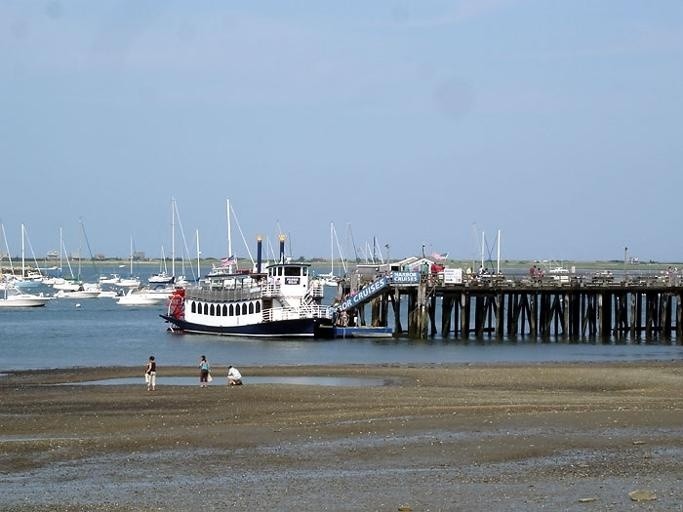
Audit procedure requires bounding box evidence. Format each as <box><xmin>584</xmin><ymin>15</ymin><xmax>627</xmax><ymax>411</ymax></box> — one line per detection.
<box><xmin>412</xmin><ymin>257</ymin><xmax>503</xmax><ymax>284</ymax></box>
<box><xmin>198</xmin><ymin>355</ymin><xmax>209</xmax><ymax>388</ymax></box>
<box><xmin>226</xmin><ymin>366</ymin><xmax>241</xmax><ymax>386</ymax></box>
<box><xmin>528</xmin><ymin>264</ymin><xmax>535</xmax><ymax>278</ymax></box>
<box><xmin>667</xmin><ymin>266</ymin><xmax>672</xmax><ymax>282</ymax></box>
<box><xmin>536</xmin><ymin>267</ymin><xmax>544</xmax><ymax>277</ymax></box>
<box><xmin>332</xmin><ymin>270</ymin><xmax>388</xmax><ymax>307</ymax></box>
<box><xmin>145</xmin><ymin>355</ymin><xmax>157</xmax><ymax>392</ymax></box>
<box><xmin>334</xmin><ymin>308</ymin><xmax>350</xmax><ymax>327</ymax></box>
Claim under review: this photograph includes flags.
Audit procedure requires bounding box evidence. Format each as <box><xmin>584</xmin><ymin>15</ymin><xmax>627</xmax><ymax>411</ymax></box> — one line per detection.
<box><xmin>220</xmin><ymin>255</ymin><xmax>236</xmax><ymax>267</ymax></box>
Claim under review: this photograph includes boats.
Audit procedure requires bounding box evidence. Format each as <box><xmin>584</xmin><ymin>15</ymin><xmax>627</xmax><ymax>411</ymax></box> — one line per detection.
<box><xmin>0</xmin><ymin>194</ymin><xmax>202</xmax><ymax>309</ymax></box>
<box><xmin>334</xmin><ymin>322</ymin><xmax>392</xmax><ymax>339</ymax></box>
<box><xmin>320</xmin><ymin>221</ymin><xmax>517</xmax><ymax>288</ymax></box>
<box><xmin>521</xmin><ymin>255</ymin><xmax>680</xmax><ymax>291</ymax></box>
<box><xmin>159</xmin><ymin>198</ymin><xmax>340</xmax><ymax>337</ymax></box>
